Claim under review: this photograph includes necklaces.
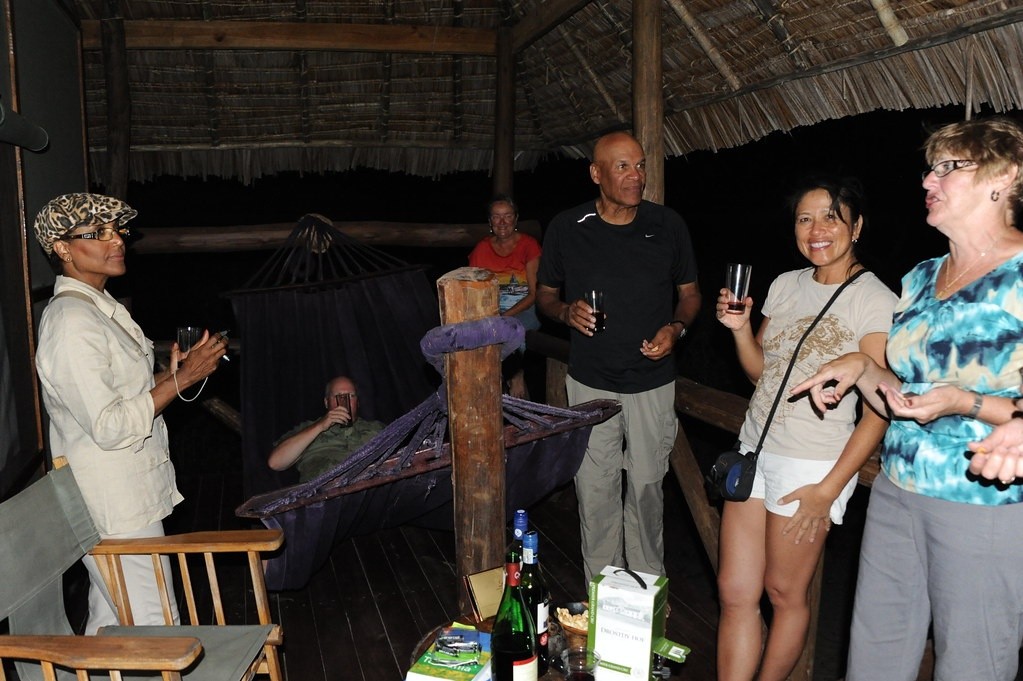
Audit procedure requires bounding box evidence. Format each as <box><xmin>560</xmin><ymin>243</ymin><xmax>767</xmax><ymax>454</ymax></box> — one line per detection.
<box><xmin>935</xmin><ymin>231</ymin><xmax>1008</xmax><ymax>299</ymax></box>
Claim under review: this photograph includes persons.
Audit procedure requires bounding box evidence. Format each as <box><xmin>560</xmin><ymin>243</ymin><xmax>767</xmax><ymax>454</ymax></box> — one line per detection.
<box><xmin>266</xmin><ymin>375</ymin><xmax>388</xmax><ymax>483</ymax></box>
<box><xmin>33</xmin><ymin>193</ymin><xmax>230</xmax><ymax>636</ymax></box>
<box><xmin>535</xmin><ymin>132</ymin><xmax>702</xmax><ymax>596</ymax></box>
<box><xmin>788</xmin><ymin>120</ymin><xmax>1023</xmax><ymax>681</ymax></box>
<box><xmin>467</xmin><ymin>196</ymin><xmax>543</xmax><ymax>400</ymax></box>
<box><xmin>717</xmin><ymin>178</ymin><xmax>900</xmax><ymax>681</ymax></box>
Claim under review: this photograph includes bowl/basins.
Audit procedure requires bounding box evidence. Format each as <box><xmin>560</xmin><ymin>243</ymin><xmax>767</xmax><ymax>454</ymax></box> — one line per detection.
<box><xmin>551</xmin><ymin>600</ymin><xmax>589</xmax><ymax>648</ymax></box>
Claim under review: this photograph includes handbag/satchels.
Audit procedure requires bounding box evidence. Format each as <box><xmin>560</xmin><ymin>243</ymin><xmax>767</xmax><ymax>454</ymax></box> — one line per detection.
<box><xmin>710</xmin><ymin>452</ymin><xmax>756</xmax><ymax>502</ymax></box>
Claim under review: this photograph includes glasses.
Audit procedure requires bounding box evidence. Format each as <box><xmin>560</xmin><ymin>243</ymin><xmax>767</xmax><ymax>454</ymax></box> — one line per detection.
<box><xmin>61</xmin><ymin>227</ymin><xmax>131</xmax><ymax>241</ymax></box>
<box><xmin>922</xmin><ymin>160</ymin><xmax>976</xmax><ymax>181</ymax></box>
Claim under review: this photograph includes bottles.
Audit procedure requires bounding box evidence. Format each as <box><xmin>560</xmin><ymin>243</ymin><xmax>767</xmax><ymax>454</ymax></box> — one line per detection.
<box><xmin>519</xmin><ymin>530</ymin><xmax>552</xmax><ymax>679</ymax></box>
<box><xmin>503</xmin><ymin>510</ymin><xmax>529</xmax><ymax>590</ymax></box>
<box><xmin>490</xmin><ymin>551</ymin><xmax>539</xmax><ymax>681</ymax></box>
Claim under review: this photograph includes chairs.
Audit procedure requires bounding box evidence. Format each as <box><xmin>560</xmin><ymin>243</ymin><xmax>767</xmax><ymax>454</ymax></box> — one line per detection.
<box><xmin>0</xmin><ymin>456</ymin><xmax>285</xmax><ymax>681</ymax></box>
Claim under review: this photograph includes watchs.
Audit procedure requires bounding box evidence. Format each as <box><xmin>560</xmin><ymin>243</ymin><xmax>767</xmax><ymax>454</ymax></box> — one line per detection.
<box><xmin>668</xmin><ymin>321</ymin><xmax>689</xmax><ymax>338</ymax></box>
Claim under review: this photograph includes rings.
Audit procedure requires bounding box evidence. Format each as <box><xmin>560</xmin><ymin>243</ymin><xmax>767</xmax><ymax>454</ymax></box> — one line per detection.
<box><xmin>1001</xmin><ymin>477</ymin><xmax>1015</xmax><ymax>484</ymax></box>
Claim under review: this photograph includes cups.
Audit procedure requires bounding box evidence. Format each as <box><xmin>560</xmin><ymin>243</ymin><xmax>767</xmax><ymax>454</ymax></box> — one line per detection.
<box><xmin>560</xmin><ymin>646</ymin><xmax>601</xmax><ymax>681</ymax></box>
<box><xmin>725</xmin><ymin>263</ymin><xmax>752</xmax><ymax>315</ymax></box>
<box><xmin>176</xmin><ymin>326</ymin><xmax>201</xmax><ymax>352</ymax></box>
<box><xmin>336</xmin><ymin>393</ymin><xmax>353</xmax><ymax>427</ymax></box>
<box><xmin>583</xmin><ymin>287</ymin><xmax>606</xmax><ymax>334</ymax></box>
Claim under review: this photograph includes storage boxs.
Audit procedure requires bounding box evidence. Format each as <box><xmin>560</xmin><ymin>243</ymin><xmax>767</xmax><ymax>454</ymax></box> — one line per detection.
<box><xmin>586</xmin><ymin>564</ymin><xmax>690</xmax><ymax>681</ymax></box>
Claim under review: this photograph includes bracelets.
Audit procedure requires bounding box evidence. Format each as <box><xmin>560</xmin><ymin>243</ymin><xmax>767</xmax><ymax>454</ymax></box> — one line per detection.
<box><xmin>1011</xmin><ymin>411</ymin><xmax>1023</xmax><ymax>419</ymax></box>
<box><xmin>963</xmin><ymin>391</ymin><xmax>982</xmax><ymax>419</ymax></box>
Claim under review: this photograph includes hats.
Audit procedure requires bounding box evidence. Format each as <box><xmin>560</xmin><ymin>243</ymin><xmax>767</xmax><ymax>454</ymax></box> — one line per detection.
<box><xmin>34</xmin><ymin>193</ymin><xmax>138</xmax><ymax>258</ymax></box>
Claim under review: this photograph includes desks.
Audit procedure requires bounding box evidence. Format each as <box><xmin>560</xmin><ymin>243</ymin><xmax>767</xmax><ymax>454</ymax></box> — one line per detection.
<box><xmin>410</xmin><ymin>614</ymin><xmax>564</xmax><ymax>681</ymax></box>
